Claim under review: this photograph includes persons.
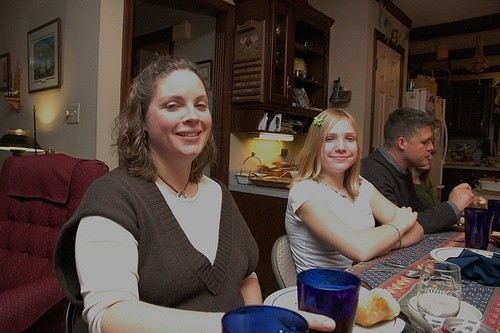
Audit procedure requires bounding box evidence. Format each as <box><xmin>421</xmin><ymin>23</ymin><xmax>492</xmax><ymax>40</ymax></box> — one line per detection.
<box><xmin>284</xmin><ymin>107</ymin><xmax>424</xmax><ymax>274</ymax></box>
<box><xmin>411</xmin><ymin>151</ymin><xmax>438</xmax><ymax>205</ymax></box>
<box><xmin>492</xmin><ymin>82</ymin><xmax>500</xmax><ymax>139</ymax></box>
<box><xmin>358</xmin><ymin>107</ymin><xmax>488</xmax><ymax>233</ymax></box>
<box><xmin>54</xmin><ymin>57</ymin><xmax>337</xmax><ymax>333</ymax></box>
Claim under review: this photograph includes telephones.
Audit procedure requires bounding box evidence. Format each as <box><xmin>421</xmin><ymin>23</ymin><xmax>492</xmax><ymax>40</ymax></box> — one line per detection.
<box><xmin>329</xmin><ymin>76</ymin><xmax>352</xmax><ymax>104</ymax></box>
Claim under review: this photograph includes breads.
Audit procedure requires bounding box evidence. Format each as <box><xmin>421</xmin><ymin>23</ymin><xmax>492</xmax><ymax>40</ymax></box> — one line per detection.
<box><xmin>354</xmin><ymin>287</ymin><xmax>401</xmax><ymax>327</ymax></box>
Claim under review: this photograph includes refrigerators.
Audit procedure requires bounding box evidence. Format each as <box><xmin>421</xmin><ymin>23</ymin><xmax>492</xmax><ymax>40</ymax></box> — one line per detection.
<box><xmin>407</xmin><ymin>88</ymin><xmax>448</xmax><ymax>204</ymax></box>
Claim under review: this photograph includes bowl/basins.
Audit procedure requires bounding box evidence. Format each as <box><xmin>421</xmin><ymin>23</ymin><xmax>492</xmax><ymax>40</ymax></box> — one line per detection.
<box><xmin>408</xmin><ymin>296</ymin><xmax>483</xmax><ymax>332</ymax></box>
<box><xmin>289</xmin><ymin>171</ymin><xmax>299</xmax><ymax>178</ymax></box>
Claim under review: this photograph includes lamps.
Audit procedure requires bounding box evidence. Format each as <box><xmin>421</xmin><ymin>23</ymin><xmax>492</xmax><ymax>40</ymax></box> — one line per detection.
<box><xmin>248</xmin><ymin>132</ymin><xmax>295</xmax><ymax>141</ymax></box>
<box><xmin>0</xmin><ymin>127</ymin><xmax>46</xmax><ymax>156</ymax></box>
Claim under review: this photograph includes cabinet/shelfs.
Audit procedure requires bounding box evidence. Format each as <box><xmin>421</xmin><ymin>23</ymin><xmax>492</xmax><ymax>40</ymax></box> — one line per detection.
<box><xmin>441</xmin><ymin>168</ymin><xmax>500</xmax><ymax>203</ymax></box>
<box><xmin>230</xmin><ymin>0</ymin><xmax>334</xmax><ymax>136</ymax></box>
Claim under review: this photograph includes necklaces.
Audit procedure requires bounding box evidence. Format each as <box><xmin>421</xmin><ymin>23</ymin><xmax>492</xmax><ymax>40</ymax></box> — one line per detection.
<box><xmin>158</xmin><ymin>176</ymin><xmax>191</xmax><ymax>199</ymax></box>
<box><xmin>321</xmin><ymin>181</ymin><xmax>347</xmax><ymax>197</ymax></box>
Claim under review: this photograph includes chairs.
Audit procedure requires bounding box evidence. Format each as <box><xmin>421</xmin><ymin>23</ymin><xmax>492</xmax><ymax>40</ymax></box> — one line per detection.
<box><xmin>0</xmin><ymin>152</ymin><xmax>109</xmax><ymax>333</ymax></box>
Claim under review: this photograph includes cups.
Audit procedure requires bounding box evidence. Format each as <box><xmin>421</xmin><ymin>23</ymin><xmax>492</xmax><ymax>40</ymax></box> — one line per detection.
<box><xmin>296</xmin><ymin>269</ymin><xmax>362</xmax><ymax>333</ymax></box>
<box><xmin>222</xmin><ymin>305</ymin><xmax>309</xmax><ymax>333</ymax></box>
<box><xmin>417</xmin><ymin>260</ymin><xmax>461</xmax><ymax>333</ymax></box>
<box><xmin>464</xmin><ymin>207</ymin><xmax>496</xmax><ymax>250</ymax></box>
<box><xmin>281</xmin><ymin>149</ymin><xmax>289</xmax><ymax>156</ymax></box>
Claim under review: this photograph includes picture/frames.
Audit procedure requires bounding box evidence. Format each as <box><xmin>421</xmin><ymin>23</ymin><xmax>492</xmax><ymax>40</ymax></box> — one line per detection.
<box><xmin>0</xmin><ymin>52</ymin><xmax>12</xmax><ymax>91</ymax></box>
<box><xmin>196</xmin><ymin>59</ymin><xmax>213</xmax><ymax>90</ymax></box>
<box><xmin>27</xmin><ymin>17</ymin><xmax>60</xmax><ymax>92</ymax></box>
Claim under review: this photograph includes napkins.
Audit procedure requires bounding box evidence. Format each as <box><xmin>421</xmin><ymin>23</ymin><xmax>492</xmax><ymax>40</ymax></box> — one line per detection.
<box><xmin>434</xmin><ymin>249</ymin><xmax>500</xmax><ymax>287</ymax></box>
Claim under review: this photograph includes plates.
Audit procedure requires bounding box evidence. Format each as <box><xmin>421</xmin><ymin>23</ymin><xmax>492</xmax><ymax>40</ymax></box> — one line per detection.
<box><xmin>264</xmin><ymin>286</ymin><xmax>405</xmax><ymax>333</ymax></box>
<box><xmin>450</xmin><ymin>217</ymin><xmax>464</xmax><ymax>231</ymax></box>
<box><xmin>272</xmin><ymin>161</ymin><xmax>288</xmax><ymax>166</ymax></box>
<box><xmin>430</xmin><ymin>247</ymin><xmax>500</xmax><ymax>262</ymax></box>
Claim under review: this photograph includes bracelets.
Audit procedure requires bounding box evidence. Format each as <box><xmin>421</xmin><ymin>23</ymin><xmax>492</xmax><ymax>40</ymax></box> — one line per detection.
<box><xmin>384</xmin><ymin>223</ymin><xmax>401</xmax><ymax>238</ymax></box>
<box><xmin>398</xmin><ymin>239</ymin><xmax>401</xmax><ymax>248</ymax></box>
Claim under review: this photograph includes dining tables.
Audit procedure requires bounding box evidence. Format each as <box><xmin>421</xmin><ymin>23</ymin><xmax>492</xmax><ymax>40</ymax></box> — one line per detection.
<box><xmin>346</xmin><ymin>187</ymin><xmax>500</xmax><ymax>333</ymax></box>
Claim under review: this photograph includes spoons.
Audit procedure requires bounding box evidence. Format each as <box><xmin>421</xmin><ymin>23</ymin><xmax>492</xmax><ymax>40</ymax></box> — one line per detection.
<box><xmin>369</xmin><ymin>268</ymin><xmax>423</xmax><ymax>278</ymax></box>
